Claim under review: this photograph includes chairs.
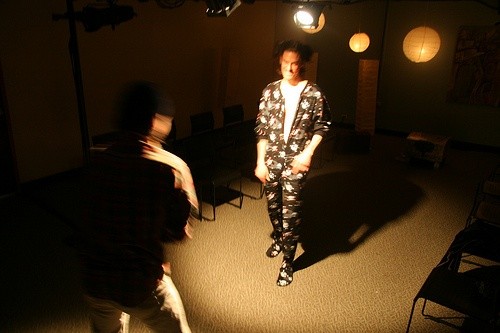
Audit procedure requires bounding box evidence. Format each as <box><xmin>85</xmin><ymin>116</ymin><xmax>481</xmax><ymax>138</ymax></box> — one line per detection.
<box><xmin>91</xmin><ymin>104</ymin><xmax>258</xmax><ymax>225</ymax></box>
<box><xmin>406</xmin><ymin>162</ymin><xmax>500</xmax><ymax>333</ymax></box>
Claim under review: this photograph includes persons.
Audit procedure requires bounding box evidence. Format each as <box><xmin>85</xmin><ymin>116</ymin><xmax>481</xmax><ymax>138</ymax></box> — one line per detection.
<box><xmin>65</xmin><ymin>82</ymin><xmax>197</xmax><ymax>333</ymax></box>
<box><xmin>254</xmin><ymin>40</ymin><xmax>333</xmax><ymax>287</ymax></box>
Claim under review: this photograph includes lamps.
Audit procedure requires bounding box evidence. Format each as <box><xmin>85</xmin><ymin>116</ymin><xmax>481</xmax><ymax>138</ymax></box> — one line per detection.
<box><xmin>348</xmin><ymin>0</ymin><xmax>370</xmax><ymax>53</ymax></box>
<box><xmin>292</xmin><ymin>0</ymin><xmax>331</xmax><ymax>34</ymax></box>
<box><xmin>403</xmin><ymin>0</ymin><xmax>442</xmax><ymax>64</ymax></box>
